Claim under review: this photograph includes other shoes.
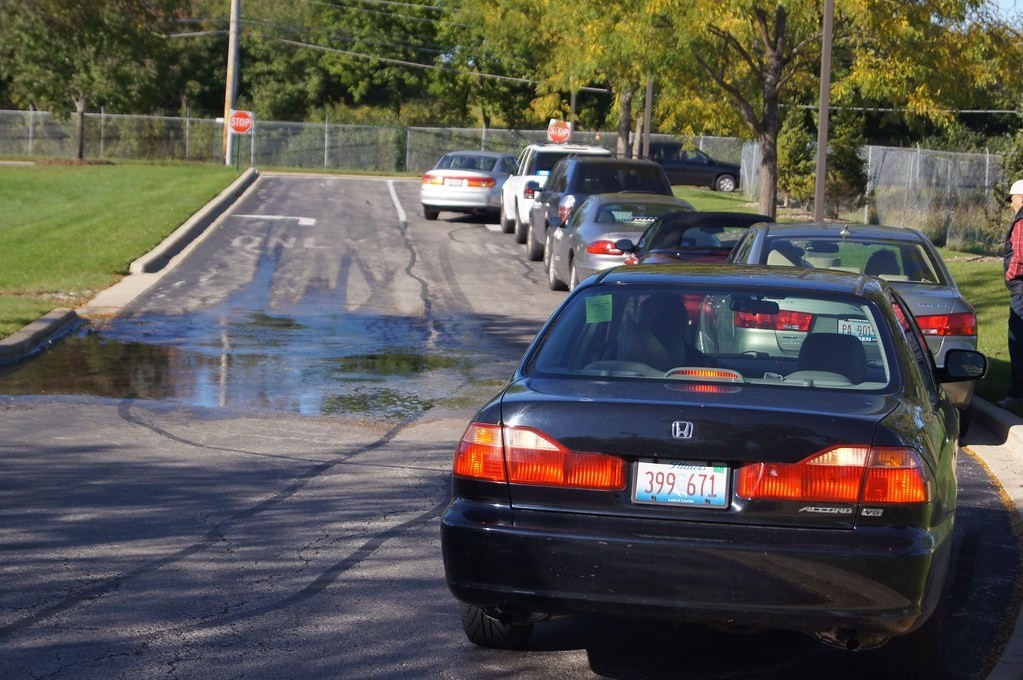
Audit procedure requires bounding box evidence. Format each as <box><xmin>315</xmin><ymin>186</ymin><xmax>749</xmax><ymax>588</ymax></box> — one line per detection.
<box><xmin>997</xmin><ymin>396</ymin><xmax>1019</xmax><ymax>408</ymax></box>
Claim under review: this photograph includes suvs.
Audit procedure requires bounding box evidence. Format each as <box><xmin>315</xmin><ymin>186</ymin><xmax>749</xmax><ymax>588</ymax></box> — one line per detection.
<box><xmin>527</xmin><ymin>152</ymin><xmax>675</xmax><ymax>274</ymax></box>
<box><xmin>500</xmin><ymin>141</ymin><xmax>612</xmax><ymax>243</ymax></box>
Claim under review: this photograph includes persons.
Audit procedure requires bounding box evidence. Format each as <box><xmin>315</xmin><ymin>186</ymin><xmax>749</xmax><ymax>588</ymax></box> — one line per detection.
<box><xmin>996</xmin><ymin>181</ymin><xmax>1023</xmax><ymax>405</ymax></box>
<box><xmin>612</xmin><ymin>294</ymin><xmax>719</xmax><ymax>371</ymax></box>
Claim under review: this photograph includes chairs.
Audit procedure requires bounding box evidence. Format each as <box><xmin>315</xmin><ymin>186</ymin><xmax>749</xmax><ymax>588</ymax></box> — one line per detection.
<box><xmin>463</xmin><ymin>157</ymin><xmax>478</xmax><ymax>169</ymax></box>
<box><xmin>612</xmin><ymin>319</ymin><xmax>686</xmax><ymax>369</ymax></box>
<box><xmin>796</xmin><ymin>333</ymin><xmax>880</xmax><ymax>384</ymax></box>
<box><xmin>865</xmin><ymin>248</ymin><xmax>901</xmax><ymax>276</ymax></box>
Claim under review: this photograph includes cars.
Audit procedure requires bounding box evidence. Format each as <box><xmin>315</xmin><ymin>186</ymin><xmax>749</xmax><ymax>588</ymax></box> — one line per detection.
<box><xmin>549</xmin><ymin>193</ymin><xmax>722</xmax><ymax>294</ymax></box>
<box><xmin>421</xmin><ymin>150</ymin><xmax>518</xmax><ymax>220</ymax></box>
<box><xmin>441</xmin><ymin>263</ymin><xmax>989</xmax><ymax>650</ymax></box>
<box><xmin>614</xmin><ymin>141</ymin><xmax>741</xmax><ymax>192</ymax></box>
<box><xmin>614</xmin><ymin>211</ymin><xmax>815</xmax><ymax>332</ymax></box>
<box><xmin>697</xmin><ymin>222</ymin><xmax>978</xmax><ymax>436</ymax></box>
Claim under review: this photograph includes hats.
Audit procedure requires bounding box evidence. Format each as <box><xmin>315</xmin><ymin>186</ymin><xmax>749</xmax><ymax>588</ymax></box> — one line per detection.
<box><xmin>1005</xmin><ymin>180</ymin><xmax>1023</xmax><ymax>203</ymax></box>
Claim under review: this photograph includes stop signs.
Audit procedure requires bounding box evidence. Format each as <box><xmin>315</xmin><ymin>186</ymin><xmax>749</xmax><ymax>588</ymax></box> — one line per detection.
<box><xmin>548</xmin><ymin>122</ymin><xmax>569</xmax><ymax>142</ymax></box>
<box><xmin>230</xmin><ymin>111</ymin><xmax>251</xmax><ymax>132</ymax></box>
